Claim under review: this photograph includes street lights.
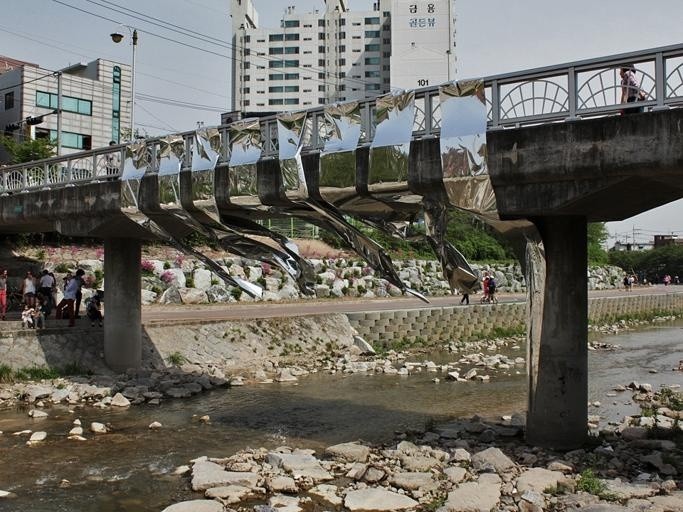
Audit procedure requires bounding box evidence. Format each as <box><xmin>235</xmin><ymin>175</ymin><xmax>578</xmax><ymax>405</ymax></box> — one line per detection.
<box><xmin>110</xmin><ymin>23</ymin><xmax>138</xmax><ymax>142</ymax></box>
<box><xmin>196</xmin><ymin>121</ymin><xmax>204</xmax><ymax>129</ymax></box>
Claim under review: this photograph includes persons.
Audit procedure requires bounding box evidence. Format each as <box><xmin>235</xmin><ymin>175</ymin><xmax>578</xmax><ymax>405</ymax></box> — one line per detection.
<box><xmin>19</xmin><ymin>268</ymin><xmax>87</xmax><ymax>330</ymax></box>
<box><xmin>0</xmin><ymin>268</ymin><xmax>9</xmax><ymax>321</ymax></box>
<box><xmin>622</xmin><ymin>274</ymin><xmax>629</xmax><ymax>292</ymax></box>
<box><xmin>481</xmin><ymin>275</ymin><xmax>498</xmax><ymax>305</ymax></box>
<box><xmin>458</xmin><ymin>294</ymin><xmax>470</xmax><ymax>305</ymax></box>
<box><xmin>86</xmin><ymin>295</ymin><xmax>103</xmax><ymax>328</ymax></box>
<box><xmin>619</xmin><ymin>62</ymin><xmax>647</xmax><ymax>113</ymax></box>
<box><xmin>627</xmin><ymin>274</ymin><xmax>634</xmax><ymax>292</ymax></box>
<box><xmin>479</xmin><ymin>277</ymin><xmax>489</xmax><ymax>302</ymax></box>
<box><xmin>106</xmin><ymin>138</ymin><xmax>124</xmax><ymax>183</ymax></box>
<box><xmin>654</xmin><ymin>272</ymin><xmax>680</xmax><ymax>287</ymax></box>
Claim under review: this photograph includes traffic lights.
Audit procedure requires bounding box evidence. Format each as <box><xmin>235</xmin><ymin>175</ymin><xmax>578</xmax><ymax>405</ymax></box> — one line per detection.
<box><xmin>5</xmin><ymin>122</ymin><xmax>21</xmax><ymax>132</ymax></box>
<box><xmin>26</xmin><ymin>117</ymin><xmax>43</xmax><ymax>125</ymax></box>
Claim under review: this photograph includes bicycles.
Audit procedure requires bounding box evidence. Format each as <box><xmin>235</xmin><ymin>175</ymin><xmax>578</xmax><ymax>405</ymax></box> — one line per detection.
<box><xmin>5</xmin><ymin>287</ymin><xmax>23</xmax><ymax>312</ymax></box>
